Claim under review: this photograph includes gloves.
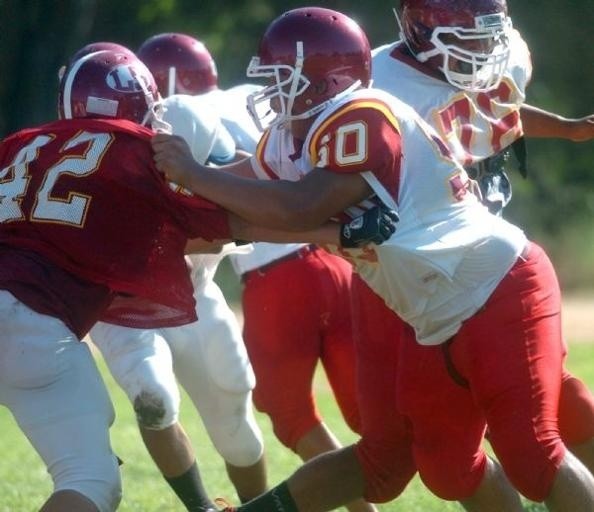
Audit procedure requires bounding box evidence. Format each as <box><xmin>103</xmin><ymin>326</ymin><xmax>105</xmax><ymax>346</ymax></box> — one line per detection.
<box><xmin>340</xmin><ymin>206</ymin><xmax>400</xmax><ymax>248</ymax></box>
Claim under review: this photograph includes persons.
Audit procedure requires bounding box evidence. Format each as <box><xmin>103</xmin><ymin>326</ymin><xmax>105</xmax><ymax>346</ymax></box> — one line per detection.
<box><xmin>138</xmin><ymin>30</ymin><xmax>363</xmax><ymax>512</ymax></box>
<box><xmin>0</xmin><ymin>40</ymin><xmax>403</xmax><ymax>512</ymax></box>
<box><xmin>86</xmin><ymin>154</ymin><xmax>272</xmax><ymax>511</ymax></box>
<box><xmin>227</xmin><ymin>0</ymin><xmax>593</xmax><ymax>512</ymax></box>
<box><xmin>147</xmin><ymin>4</ymin><xmax>594</xmax><ymax>512</ymax></box>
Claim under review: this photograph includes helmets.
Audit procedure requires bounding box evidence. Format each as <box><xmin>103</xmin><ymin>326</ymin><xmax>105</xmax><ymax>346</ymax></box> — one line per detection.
<box><xmin>393</xmin><ymin>0</ymin><xmax>515</xmax><ymax>93</ymax></box>
<box><xmin>245</xmin><ymin>4</ymin><xmax>373</xmax><ymax>130</ymax></box>
<box><xmin>58</xmin><ymin>33</ymin><xmax>219</xmax><ymax>135</ymax></box>
